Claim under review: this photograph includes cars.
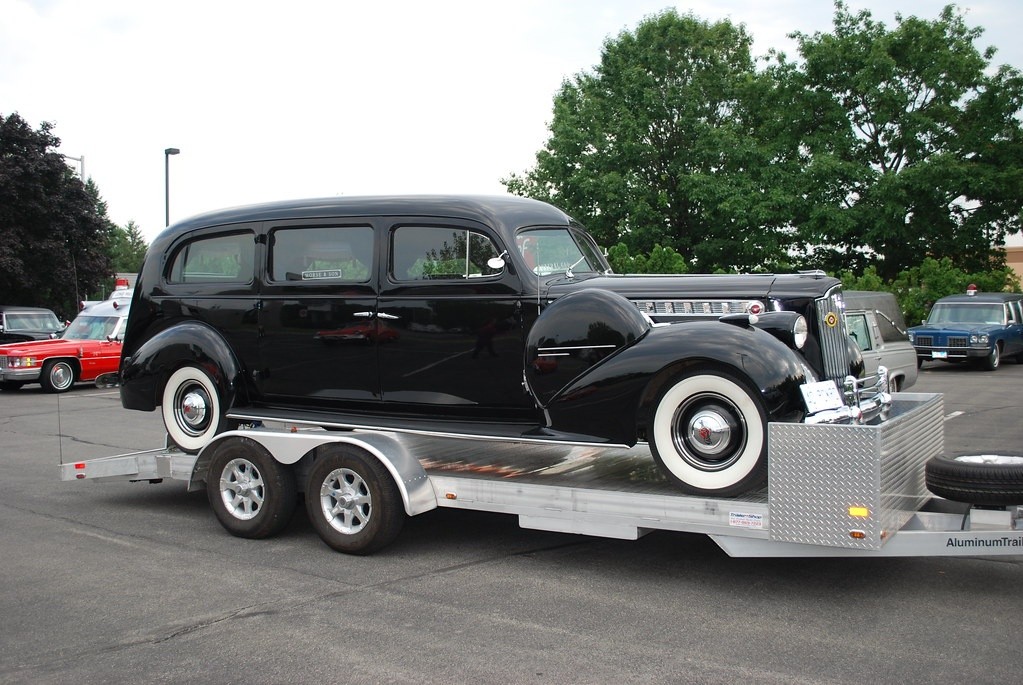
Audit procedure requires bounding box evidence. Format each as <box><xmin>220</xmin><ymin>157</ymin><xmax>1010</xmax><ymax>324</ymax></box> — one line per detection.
<box><xmin>118</xmin><ymin>194</ymin><xmax>893</xmax><ymax>498</ymax></box>
<box><xmin>0</xmin><ymin>306</ymin><xmax>63</xmax><ymax>344</ymax></box>
<box><xmin>906</xmin><ymin>284</ymin><xmax>1023</xmax><ymax>371</ymax></box>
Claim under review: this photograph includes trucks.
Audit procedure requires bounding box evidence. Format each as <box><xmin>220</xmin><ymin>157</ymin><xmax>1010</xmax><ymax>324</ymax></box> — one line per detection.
<box><xmin>0</xmin><ymin>272</ymin><xmax>236</xmax><ymax>395</ymax></box>
<box><xmin>842</xmin><ymin>291</ymin><xmax>919</xmax><ymax>394</ymax></box>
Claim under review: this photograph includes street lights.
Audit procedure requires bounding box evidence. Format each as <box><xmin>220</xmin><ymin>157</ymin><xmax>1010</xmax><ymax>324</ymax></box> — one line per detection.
<box><xmin>165</xmin><ymin>148</ymin><xmax>180</xmax><ymax>227</ymax></box>
